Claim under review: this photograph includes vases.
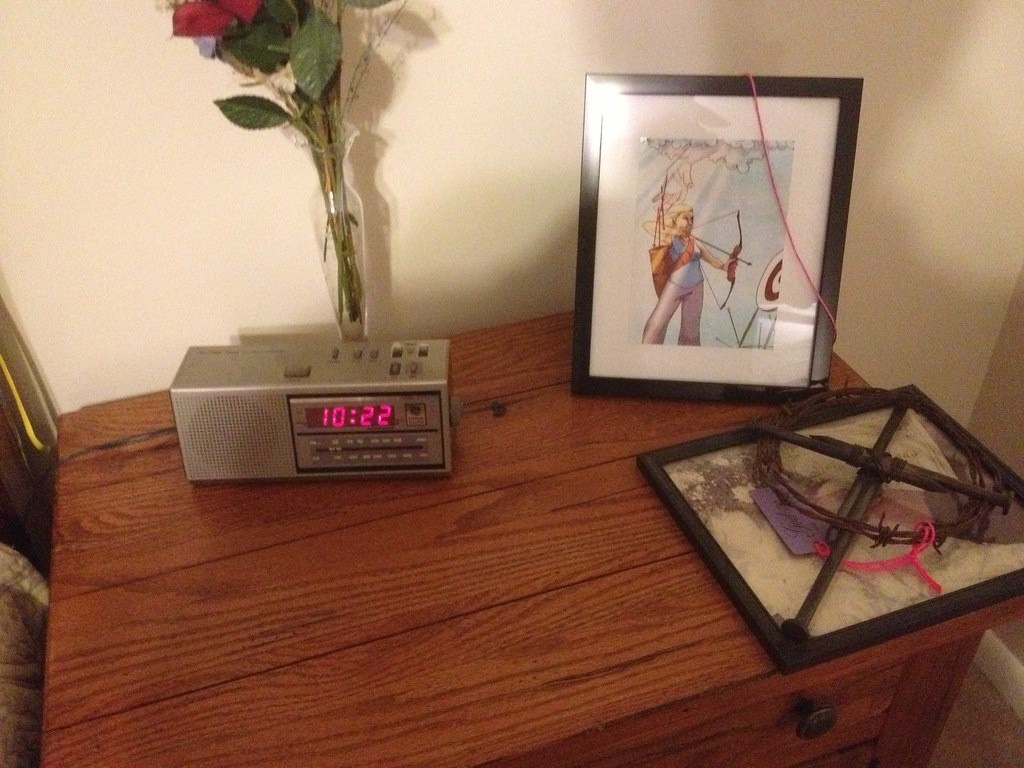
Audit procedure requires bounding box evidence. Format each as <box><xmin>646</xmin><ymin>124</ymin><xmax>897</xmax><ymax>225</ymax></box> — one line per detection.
<box><xmin>283</xmin><ymin>122</ymin><xmax>366</xmax><ymax>341</ymax></box>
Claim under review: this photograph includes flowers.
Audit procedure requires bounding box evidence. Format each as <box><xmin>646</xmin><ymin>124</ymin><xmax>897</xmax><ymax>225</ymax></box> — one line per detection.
<box><xmin>162</xmin><ymin>0</ymin><xmax>407</xmax><ymax>149</ymax></box>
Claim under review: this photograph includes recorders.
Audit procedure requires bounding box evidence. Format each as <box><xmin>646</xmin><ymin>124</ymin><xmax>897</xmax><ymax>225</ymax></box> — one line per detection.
<box><xmin>171</xmin><ymin>335</ymin><xmax>453</xmax><ymax>487</ymax></box>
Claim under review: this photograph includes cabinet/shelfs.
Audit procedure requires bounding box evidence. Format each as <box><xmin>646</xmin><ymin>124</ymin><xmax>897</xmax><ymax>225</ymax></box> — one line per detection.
<box><xmin>38</xmin><ymin>316</ymin><xmax>1023</xmax><ymax>767</ymax></box>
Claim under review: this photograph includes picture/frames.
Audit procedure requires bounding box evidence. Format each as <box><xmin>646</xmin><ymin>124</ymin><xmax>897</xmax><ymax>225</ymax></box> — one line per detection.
<box><xmin>571</xmin><ymin>73</ymin><xmax>865</xmax><ymax>402</ymax></box>
<box><xmin>634</xmin><ymin>384</ymin><xmax>1024</xmax><ymax>675</ymax></box>
